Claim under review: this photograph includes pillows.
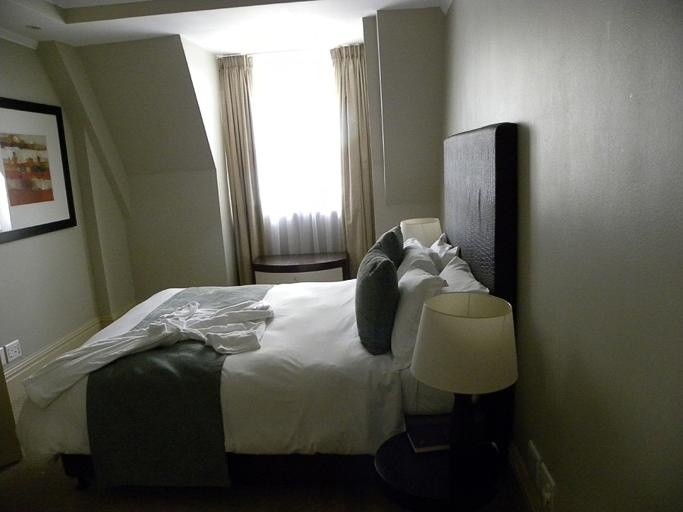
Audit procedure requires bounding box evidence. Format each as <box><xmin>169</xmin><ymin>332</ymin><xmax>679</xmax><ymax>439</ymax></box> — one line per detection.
<box><xmin>354</xmin><ymin>225</ymin><xmax>487</xmax><ymax>365</ymax></box>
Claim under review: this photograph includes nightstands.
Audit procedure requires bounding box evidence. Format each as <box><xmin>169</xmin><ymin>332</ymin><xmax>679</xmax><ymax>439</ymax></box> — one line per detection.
<box><xmin>372</xmin><ymin>429</ymin><xmax>511</xmax><ymax>512</ymax></box>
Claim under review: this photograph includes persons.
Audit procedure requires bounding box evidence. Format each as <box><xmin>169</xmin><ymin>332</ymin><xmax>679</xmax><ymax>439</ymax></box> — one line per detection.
<box><xmin>6</xmin><ymin>152</ymin><xmax>43</xmax><ymax>181</ymax></box>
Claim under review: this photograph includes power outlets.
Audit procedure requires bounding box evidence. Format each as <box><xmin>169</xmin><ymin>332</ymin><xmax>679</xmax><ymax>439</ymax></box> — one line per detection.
<box><xmin>4</xmin><ymin>339</ymin><xmax>24</xmax><ymax>362</ymax></box>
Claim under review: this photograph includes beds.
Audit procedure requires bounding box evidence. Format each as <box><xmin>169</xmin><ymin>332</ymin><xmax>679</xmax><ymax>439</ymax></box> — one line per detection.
<box><xmin>14</xmin><ymin>121</ymin><xmax>519</xmax><ymax>496</ymax></box>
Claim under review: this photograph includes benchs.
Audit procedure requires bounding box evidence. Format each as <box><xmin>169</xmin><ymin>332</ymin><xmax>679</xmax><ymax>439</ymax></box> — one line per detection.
<box><xmin>253</xmin><ymin>252</ymin><xmax>350</xmax><ymax>285</ymax></box>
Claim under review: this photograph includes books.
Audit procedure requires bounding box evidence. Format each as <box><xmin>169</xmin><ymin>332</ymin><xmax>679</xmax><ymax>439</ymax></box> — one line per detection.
<box><xmin>406</xmin><ymin>424</ymin><xmax>451</xmax><ymax>454</ymax></box>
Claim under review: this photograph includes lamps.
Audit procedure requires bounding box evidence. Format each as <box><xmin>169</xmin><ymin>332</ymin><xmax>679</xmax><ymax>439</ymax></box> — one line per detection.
<box><xmin>400</xmin><ymin>217</ymin><xmax>442</xmax><ymax>247</ymax></box>
<box><xmin>414</xmin><ymin>291</ymin><xmax>520</xmax><ymax>468</ymax></box>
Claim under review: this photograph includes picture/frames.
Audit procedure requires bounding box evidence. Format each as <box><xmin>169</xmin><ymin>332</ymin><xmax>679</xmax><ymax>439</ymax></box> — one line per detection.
<box><xmin>0</xmin><ymin>95</ymin><xmax>79</xmax><ymax>246</ymax></box>
